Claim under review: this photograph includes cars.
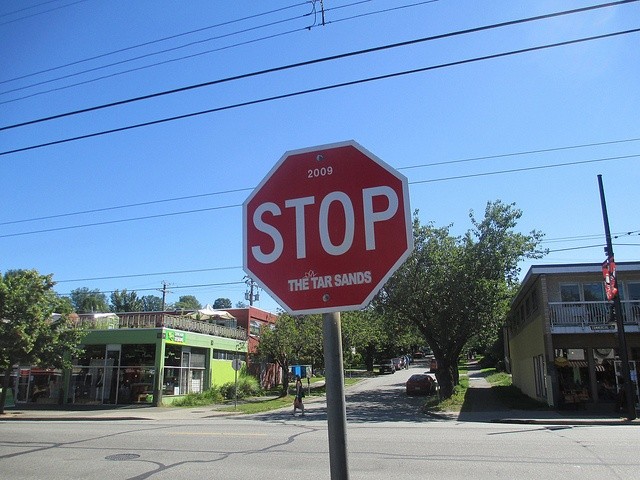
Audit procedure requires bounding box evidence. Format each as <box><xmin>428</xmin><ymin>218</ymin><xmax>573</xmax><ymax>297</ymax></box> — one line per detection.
<box><xmin>393</xmin><ymin>357</ymin><xmax>405</xmax><ymax>370</ymax></box>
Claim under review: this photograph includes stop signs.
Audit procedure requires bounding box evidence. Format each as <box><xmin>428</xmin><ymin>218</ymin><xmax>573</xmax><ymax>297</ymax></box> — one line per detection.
<box><xmin>241</xmin><ymin>138</ymin><xmax>415</xmax><ymax>318</ymax></box>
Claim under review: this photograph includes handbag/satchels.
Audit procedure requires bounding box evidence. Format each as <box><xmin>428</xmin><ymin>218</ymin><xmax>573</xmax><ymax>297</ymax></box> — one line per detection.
<box><xmin>294</xmin><ymin>394</ymin><xmax>300</xmax><ymax>408</ymax></box>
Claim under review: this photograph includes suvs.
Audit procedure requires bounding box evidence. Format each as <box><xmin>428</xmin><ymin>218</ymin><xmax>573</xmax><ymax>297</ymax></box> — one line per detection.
<box><xmin>379</xmin><ymin>359</ymin><xmax>396</xmax><ymax>374</ymax></box>
<box><xmin>406</xmin><ymin>374</ymin><xmax>437</xmax><ymax>396</ymax></box>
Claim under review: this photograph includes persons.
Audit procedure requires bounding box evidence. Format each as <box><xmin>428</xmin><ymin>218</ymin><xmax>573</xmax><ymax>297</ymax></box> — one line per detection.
<box><xmin>95</xmin><ymin>368</ymin><xmax>104</xmax><ymax>401</ymax></box>
<box><xmin>289</xmin><ymin>375</ymin><xmax>306</xmax><ymax>416</ymax></box>
<box><xmin>469</xmin><ymin>351</ymin><xmax>472</xmax><ymax>359</ymax></box>
<box><xmin>473</xmin><ymin>350</ymin><xmax>477</xmax><ymax>359</ymax></box>
<box><xmin>405</xmin><ymin>356</ymin><xmax>409</xmax><ymax>370</ymax></box>
<box><xmin>83</xmin><ymin>369</ymin><xmax>93</xmax><ymax>401</ymax></box>
